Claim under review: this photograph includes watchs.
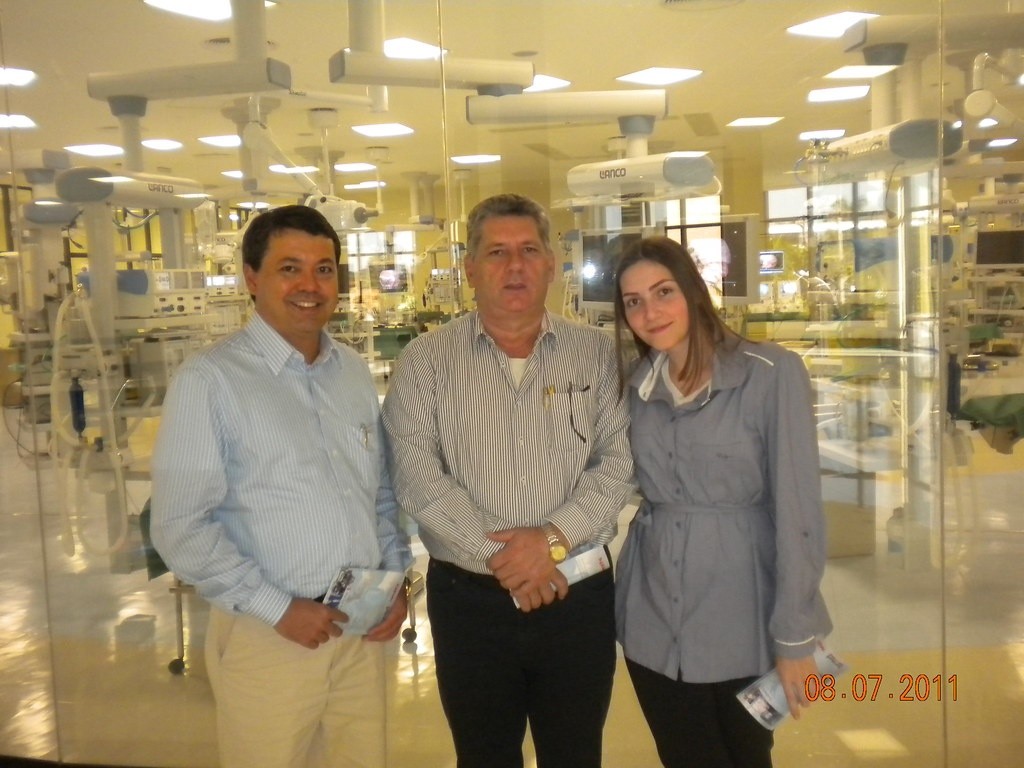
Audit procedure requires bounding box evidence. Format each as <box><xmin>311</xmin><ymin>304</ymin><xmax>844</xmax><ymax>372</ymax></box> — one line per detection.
<box><xmin>542</xmin><ymin>523</ymin><xmax>567</xmax><ymax>564</ymax></box>
<box><xmin>400</xmin><ymin>578</ymin><xmax>412</xmax><ymax>604</ymax></box>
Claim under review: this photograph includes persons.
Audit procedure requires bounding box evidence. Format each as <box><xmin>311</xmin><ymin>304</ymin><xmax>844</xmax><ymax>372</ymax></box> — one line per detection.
<box><xmin>148</xmin><ymin>205</ymin><xmax>413</xmax><ymax>768</ymax></box>
<box><xmin>381</xmin><ymin>191</ymin><xmax>635</xmax><ymax>768</ymax></box>
<box><xmin>617</xmin><ymin>235</ymin><xmax>833</xmax><ymax>768</ymax></box>
